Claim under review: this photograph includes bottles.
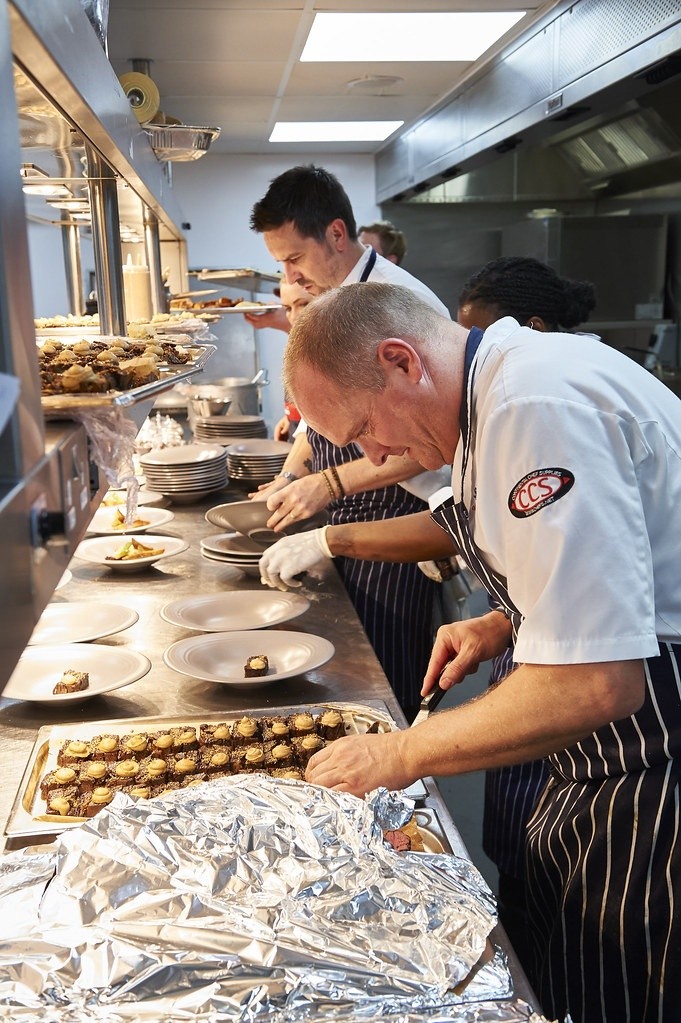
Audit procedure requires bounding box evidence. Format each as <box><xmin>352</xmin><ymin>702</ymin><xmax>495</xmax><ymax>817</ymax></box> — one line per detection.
<box><xmin>122</xmin><ymin>253</ymin><xmax>151</xmax><ymax>323</ymax></box>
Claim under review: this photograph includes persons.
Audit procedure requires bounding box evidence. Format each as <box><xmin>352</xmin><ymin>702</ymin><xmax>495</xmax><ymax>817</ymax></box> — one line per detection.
<box><xmin>246</xmin><ymin>163</ymin><xmax>459</xmax><ymax>737</ymax></box>
<box><xmin>245</xmin><ymin>222</ymin><xmax>408</xmax><ymax>335</ymax></box>
<box><xmin>278</xmin><ymin>284</ymin><xmax>681</xmax><ymax>1023</ymax></box>
<box><xmin>273</xmin><ymin>271</ymin><xmax>327</xmax><ymax>444</ymax></box>
<box><xmin>254</xmin><ymin>253</ymin><xmax>602</xmax><ymax>950</ymax></box>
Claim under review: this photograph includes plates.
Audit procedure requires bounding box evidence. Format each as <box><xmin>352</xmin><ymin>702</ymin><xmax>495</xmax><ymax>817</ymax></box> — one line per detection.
<box><xmin>2</xmin><ymin>414</ymin><xmax>336</xmax><ymax>707</ymax></box>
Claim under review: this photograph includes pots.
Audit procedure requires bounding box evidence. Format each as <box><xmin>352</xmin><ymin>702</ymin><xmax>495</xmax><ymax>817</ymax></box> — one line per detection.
<box><xmin>184</xmin><ymin>367</ymin><xmax>271</xmax><ymax>435</ymax></box>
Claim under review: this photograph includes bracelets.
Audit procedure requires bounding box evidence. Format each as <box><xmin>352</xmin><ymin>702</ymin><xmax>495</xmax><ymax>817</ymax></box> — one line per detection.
<box><xmin>320</xmin><ymin>466</ymin><xmax>346</xmax><ymax>503</ymax></box>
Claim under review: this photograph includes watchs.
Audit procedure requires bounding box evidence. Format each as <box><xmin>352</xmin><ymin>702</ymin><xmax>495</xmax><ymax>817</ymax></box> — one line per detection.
<box><xmin>279</xmin><ymin>472</ymin><xmax>300</xmax><ymax>482</ymax></box>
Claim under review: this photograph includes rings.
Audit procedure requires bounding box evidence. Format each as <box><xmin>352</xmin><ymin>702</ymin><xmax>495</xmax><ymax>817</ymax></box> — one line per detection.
<box><xmin>290</xmin><ymin>511</ymin><xmax>298</xmax><ymax>521</ymax></box>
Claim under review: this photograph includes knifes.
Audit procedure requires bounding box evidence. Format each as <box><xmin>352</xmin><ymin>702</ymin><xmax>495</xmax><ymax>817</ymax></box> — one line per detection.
<box><xmin>410</xmin><ymin>668</ymin><xmax>446</xmax><ymax>728</ymax></box>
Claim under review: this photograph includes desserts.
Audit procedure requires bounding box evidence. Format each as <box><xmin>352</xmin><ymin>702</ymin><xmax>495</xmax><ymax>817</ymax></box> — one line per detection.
<box><xmin>52</xmin><ymin>669</ymin><xmax>89</xmax><ymax>693</ymax></box>
<box><xmin>243</xmin><ymin>655</ymin><xmax>270</xmax><ymax>678</ymax></box>
<box><xmin>39</xmin><ymin>708</ymin><xmax>346</xmax><ymax>817</ymax></box>
<box><xmin>36</xmin><ymin>297</ymin><xmax>241</xmax><ymax>397</ymax></box>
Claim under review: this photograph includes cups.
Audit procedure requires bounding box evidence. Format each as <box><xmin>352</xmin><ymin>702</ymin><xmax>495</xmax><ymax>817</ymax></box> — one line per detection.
<box><xmin>186</xmin><ymin>397</ymin><xmax>232</xmax><ymax>430</ymax></box>
